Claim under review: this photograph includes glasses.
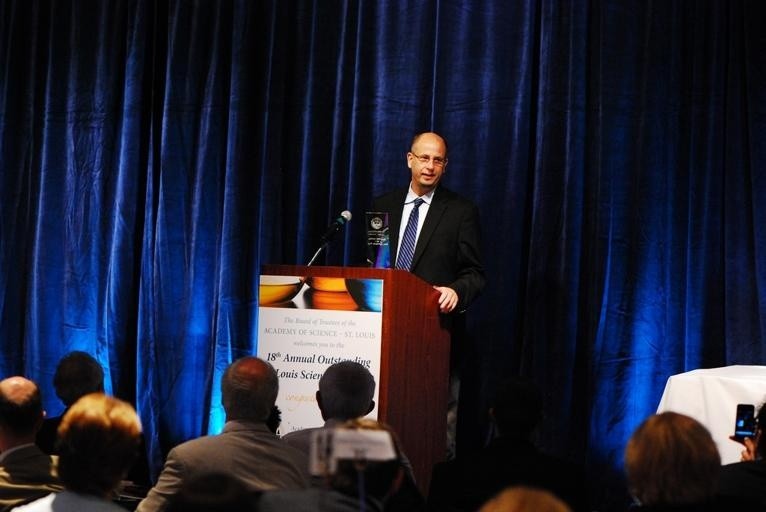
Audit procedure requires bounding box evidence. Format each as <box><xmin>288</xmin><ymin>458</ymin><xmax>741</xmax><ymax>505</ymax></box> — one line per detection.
<box><xmin>412</xmin><ymin>153</ymin><xmax>445</xmax><ymax>167</ymax></box>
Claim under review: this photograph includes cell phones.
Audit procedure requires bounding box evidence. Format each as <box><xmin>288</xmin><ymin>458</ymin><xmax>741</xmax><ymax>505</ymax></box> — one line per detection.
<box><xmin>731</xmin><ymin>402</ymin><xmax>755</xmax><ymax>439</ymax></box>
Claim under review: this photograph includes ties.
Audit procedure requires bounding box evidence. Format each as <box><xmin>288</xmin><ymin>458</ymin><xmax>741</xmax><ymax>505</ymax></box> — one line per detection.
<box><xmin>396</xmin><ymin>199</ymin><xmax>424</xmax><ymax>271</ymax></box>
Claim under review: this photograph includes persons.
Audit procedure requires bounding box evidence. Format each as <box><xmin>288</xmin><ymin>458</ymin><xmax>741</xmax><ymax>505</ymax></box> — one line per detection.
<box><xmin>366</xmin><ymin>132</ymin><xmax>490</xmax><ymax>464</ymax></box>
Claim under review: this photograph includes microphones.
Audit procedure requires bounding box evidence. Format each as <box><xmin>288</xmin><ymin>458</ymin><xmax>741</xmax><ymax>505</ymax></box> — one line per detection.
<box><xmin>322</xmin><ymin>209</ymin><xmax>353</xmax><ymax>241</ymax></box>
